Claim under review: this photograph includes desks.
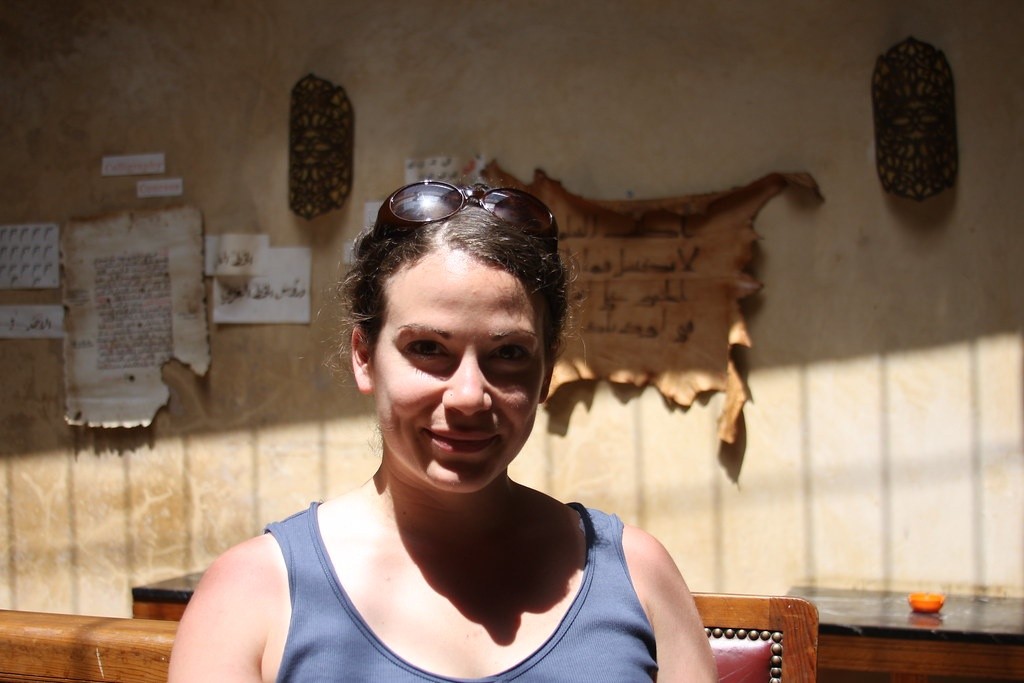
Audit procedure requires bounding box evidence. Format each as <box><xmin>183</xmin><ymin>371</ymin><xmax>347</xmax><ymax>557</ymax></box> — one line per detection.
<box><xmin>131</xmin><ymin>571</ymin><xmax>210</xmax><ymax>621</ymax></box>
<box><xmin>784</xmin><ymin>586</ymin><xmax>1024</xmax><ymax>683</ymax></box>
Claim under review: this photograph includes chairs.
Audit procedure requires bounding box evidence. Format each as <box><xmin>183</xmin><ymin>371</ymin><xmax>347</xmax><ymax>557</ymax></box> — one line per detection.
<box><xmin>690</xmin><ymin>593</ymin><xmax>819</xmax><ymax>683</ymax></box>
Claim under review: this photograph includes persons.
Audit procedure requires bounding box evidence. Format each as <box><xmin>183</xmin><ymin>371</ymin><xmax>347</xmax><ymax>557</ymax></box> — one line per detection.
<box><xmin>167</xmin><ymin>180</ymin><xmax>719</xmax><ymax>683</ymax></box>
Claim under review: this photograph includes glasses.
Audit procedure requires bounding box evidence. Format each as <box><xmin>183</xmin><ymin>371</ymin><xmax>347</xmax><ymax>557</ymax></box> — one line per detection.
<box><xmin>375</xmin><ymin>179</ymin><xmax>559</xmax><ymax>246</ymax></box>
